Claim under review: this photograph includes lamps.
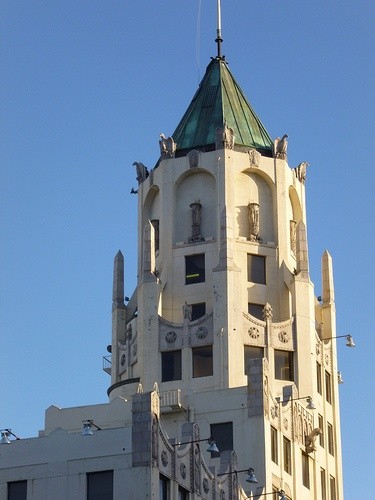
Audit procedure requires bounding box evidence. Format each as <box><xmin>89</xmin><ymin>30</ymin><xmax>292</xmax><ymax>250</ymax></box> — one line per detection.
<box><xmin>216</xmin><ymin>466</ymin><xmax>257</xmax><ymax>483</ymax></box>
<box><xmin>172</xmin><ymin>437</ymin><xmax>219</xmax><ymax>452</ymax></box>
<box><xmin>277</xmin><ymin>396</ymin><xmax>317</xmax><ymax>409</ymax></box>
<box><xmin>81</xmin><ymin>419</ymin><xmax>101</xmax><ymax>436</ymax></box>
<box><xmin>338</xmin><ymin>371</ymin><xmax>344</xmax><ymax>384</ymax></box>
<box><xmin>0</xmin><ymin>428</ymin><xmax>22</xmax><ymax>445</ymax></box>
<box><xmin>248</xmin><ymin>489</ymin><xmax>289</xmax><ymax>500</ymax></box>
<box><xmin>321</xmin><ymin>335</ymin><xmax>355</xmax><ymax>347</ymax></box>
